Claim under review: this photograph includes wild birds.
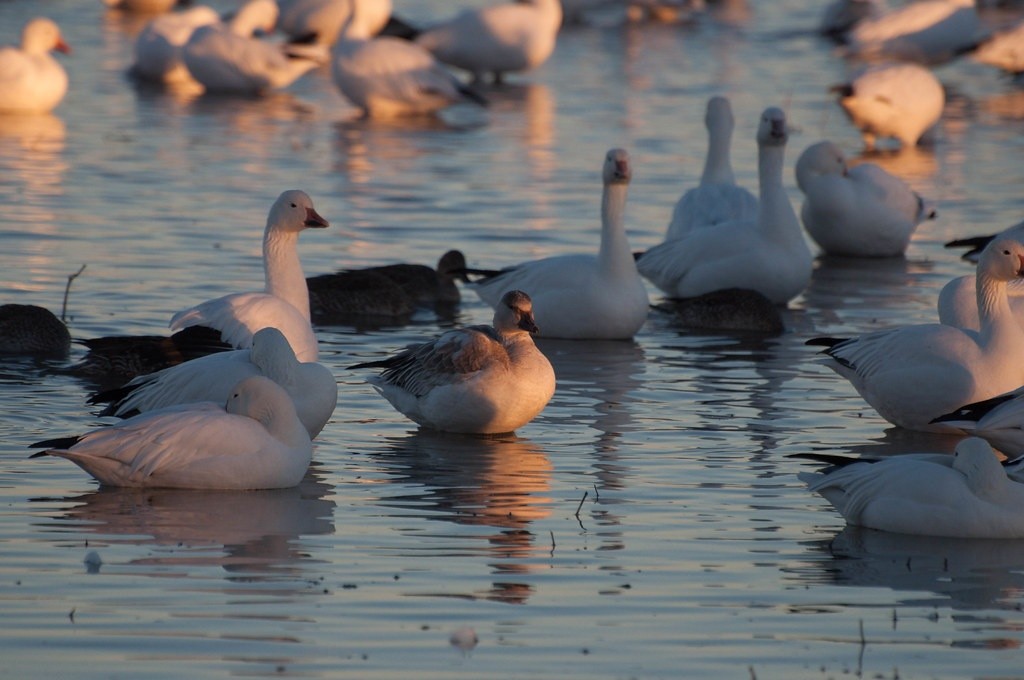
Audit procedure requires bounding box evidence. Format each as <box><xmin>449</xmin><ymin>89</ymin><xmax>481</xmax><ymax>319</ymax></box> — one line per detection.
<box><xmin>465</xmin><ymin>148</ymin><xmax>648</xmax><ymax>342</ymax></box>
<box><xmin>0</xmin><ymin>0</ymin><xmax>1023</xmax><ymax>160</ymax></box>
<box><xmin>308</xmin><ymin>251</ymin><xmax>475</xmax><ymax>325</ymax></box>
<box><xmin>782</xmin><ymin>221</ymin><xmax>1024</xmax><ymax>542</ymax></box>
<box><xmin>343</xmin><ymin>290</ymin><xmax>555</xmax><ymax>435</ymax></box>
<box><xmin>630</xmin><ymin>95</ymin><xmax>936</xmax><ymax>316</ymax></box>
<box><xmin>0</xmin><ymin>190</ymin><xmax>339</xmax><ymax>494</ymax></box>
